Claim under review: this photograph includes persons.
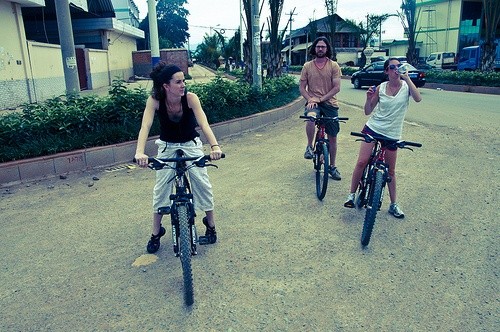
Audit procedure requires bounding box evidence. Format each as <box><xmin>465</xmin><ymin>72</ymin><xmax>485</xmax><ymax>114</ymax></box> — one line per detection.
<box><xmin>344</xmin><ymin>58</ymin><xmax>423</xmax><ymax>218</ymax></box>
<box><xmin>135</xmin><ymin>61</ymin><xmax>223</xmax><ymax>254</ymax></box>
<box><xmin>299</xmin><ymin>36</ymin><xmax>342</xmax><ymax>180</ymax></box>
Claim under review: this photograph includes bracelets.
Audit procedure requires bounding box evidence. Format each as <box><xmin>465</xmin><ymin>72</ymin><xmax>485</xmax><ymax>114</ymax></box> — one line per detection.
<box><xmin>319</xmin><ymin>98</ymin><xmax>321</xmax><ymax>102</ymax></box>
<box><xmin>211</xmin><ymin>144</ymin><xmax>220</xmax><ymax>148</ymax></box>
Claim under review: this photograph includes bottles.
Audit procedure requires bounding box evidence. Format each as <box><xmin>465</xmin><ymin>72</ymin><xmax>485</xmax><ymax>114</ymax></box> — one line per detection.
<box><xmin>436</xmin><ymin>87</ymin><xmax>442</xmax><ymax>91</ymax></box>
<box><xmin>396</xmin><ymin>63</ymin><xmax>411</xmax><ymax>77</ymax></box>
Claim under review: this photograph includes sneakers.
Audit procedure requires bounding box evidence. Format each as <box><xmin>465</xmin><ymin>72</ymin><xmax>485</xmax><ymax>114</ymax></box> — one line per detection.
<box><xmin>344</xmin><ymin>194</ymin><xmax>355</xmax><ymax>208</ymax></box>
<box><xmin>328</xmin><ymin>166</ymin><xmax>341</xmax><ymax>180</ymax></box>
<box><xmin>389</xmin><ymin>204</ymin><xmax>405</xmax><ymax>218</ymax></box>
<box><xmin>304</xmin><ymin>146</ymin><xmax>314</xmax><ymax>159</ymax></box>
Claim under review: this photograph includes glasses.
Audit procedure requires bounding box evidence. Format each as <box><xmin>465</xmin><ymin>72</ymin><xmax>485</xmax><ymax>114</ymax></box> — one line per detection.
<box><xmin>386</xmin><ymin>64</ymin><xmax>403</xmax><ymax>70</ymax></box>
<box><xmin>316</xmin><ymin>46</ymin><xmax>327</xmax><ymax>49</ymax></box>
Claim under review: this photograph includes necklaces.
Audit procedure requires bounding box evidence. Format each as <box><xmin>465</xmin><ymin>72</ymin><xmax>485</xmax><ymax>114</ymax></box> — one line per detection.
<box><xmin>390</xmin><ymin>82</ymin><xmax>402</xmax><ymax>96</ymax></box>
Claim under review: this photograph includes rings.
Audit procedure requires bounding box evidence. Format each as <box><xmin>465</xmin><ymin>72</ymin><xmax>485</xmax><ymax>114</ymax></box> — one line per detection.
<box><xmin>142</xmin><ymin>161</ymin><xmax>146</xmax><ymax>163</ymax></box>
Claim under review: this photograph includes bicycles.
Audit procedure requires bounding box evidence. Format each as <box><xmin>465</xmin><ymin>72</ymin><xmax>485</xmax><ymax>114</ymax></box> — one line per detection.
<box><xmin>133</xmin><ymin>149</ymin><xmax>225</xmax><ymax>307</ymax></box>
<box><xmin>299</xmin><ymin>110</ymin><xmax>349</xmax><ymax>200</ymax></box>
<box><xmin>350</xmin><ymin>132</ymin><xmax>422</xmax><ymax>246</ymax></box>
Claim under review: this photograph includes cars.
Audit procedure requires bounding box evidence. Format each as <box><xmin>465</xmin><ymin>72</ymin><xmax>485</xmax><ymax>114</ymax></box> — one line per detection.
<box><xmin>188</xmin><ymin>58</ymin><xmax>199</xmax><ymax>63</ymax></box>
<box><xmin>351</xmin><ymin>61</ymin><xmax>426</xmax><ymax>94</ymax></box>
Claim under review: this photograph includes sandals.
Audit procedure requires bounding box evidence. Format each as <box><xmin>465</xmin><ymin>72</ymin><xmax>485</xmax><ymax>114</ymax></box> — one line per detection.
<box><xmin>203</xmin><ymin>217</ymin><xmax>217</xmax><ymax>244</ymax></box>
<box><xmin>147</xmin><ymin>224</ymin><xmax>166</xmax><ymax>254</ymax></box>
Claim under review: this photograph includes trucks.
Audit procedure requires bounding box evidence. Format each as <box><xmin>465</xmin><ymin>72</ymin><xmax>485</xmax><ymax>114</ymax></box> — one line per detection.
<box><xmin>457</xmin><ymin>38</ymin><xmax>500</xmax><ymax>72</ymax></box>
<box><xmin>425</xmin><ymin>51</ymin><xmax>457</xmax><ymax>72</ymax></box>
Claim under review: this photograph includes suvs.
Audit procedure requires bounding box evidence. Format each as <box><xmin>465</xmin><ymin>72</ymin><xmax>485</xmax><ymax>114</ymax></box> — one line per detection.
<box><xmin>370</xmin><ymin>56</ymin><xmax>398</xmax><ymax>65</ymax></box>
<box><xmin>386</xmin><ymin>56</ymin><xmax>408</xmax><ymax>67</ymax></box>
